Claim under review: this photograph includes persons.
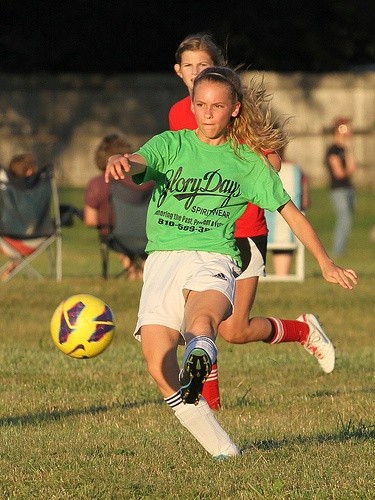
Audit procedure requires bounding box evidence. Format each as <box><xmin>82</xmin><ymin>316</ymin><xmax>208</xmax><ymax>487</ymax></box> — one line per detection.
<box><xmin>264</xmin><ymin>123</ymin><xmax>310</xmax><ymax>276</ymax></box>
<box><xmin>105</xmin><ymin>66</ymin><xmax>357</xmax><ymax>459</ymax></box>
<box><xmin>327</xmin><ymin>118</ymin><xmax>357</xmax><ymax>257</ymax></box>
<box><xmin>84</xmin><ymin>134</ymin><xmax>154</xmax><ymax>283</ymax></box>
<box><xmin>0</xmin><ymin>154</ymin><xmax>48</xmax><ymax>280</ymax></box>
<box><xmin>169</xmin><ymin>34</ymin><xmax>336</xmax><ymax>414</ymax></box>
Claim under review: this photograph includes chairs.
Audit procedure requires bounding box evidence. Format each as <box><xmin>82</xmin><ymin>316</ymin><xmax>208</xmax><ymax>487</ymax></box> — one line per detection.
<box><xmin>0</xmin><ymin>162</ymin><xmax>64</xmax><ymax>282</ymax></box>
<box><xmin>100</xmin><ymin>177</ymin><xmax>155</xmax><ymax>280</ymax></box>
<box><xmin>257</xmin><ymin>162</ymin><xmax>306</xmax><ymax>283</ymax></box>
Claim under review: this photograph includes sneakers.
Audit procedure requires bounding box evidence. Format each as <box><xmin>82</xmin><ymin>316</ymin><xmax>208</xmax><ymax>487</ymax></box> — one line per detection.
<box><xmin>295</xmin><ymin>312</ymin><xmax>337</xmax><ymax>374</ymax></box>
<box><xmin>178</xmin><ymin>348</ymin><xmax>213</xmax><ymax>405</ymax></box>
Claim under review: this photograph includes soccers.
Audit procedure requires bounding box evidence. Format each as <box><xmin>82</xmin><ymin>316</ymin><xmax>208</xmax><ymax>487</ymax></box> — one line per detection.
<box><xmin>51</xmin><ymin>293</ymin><xmax>116</xmax><ymax>360</ymax></box>
